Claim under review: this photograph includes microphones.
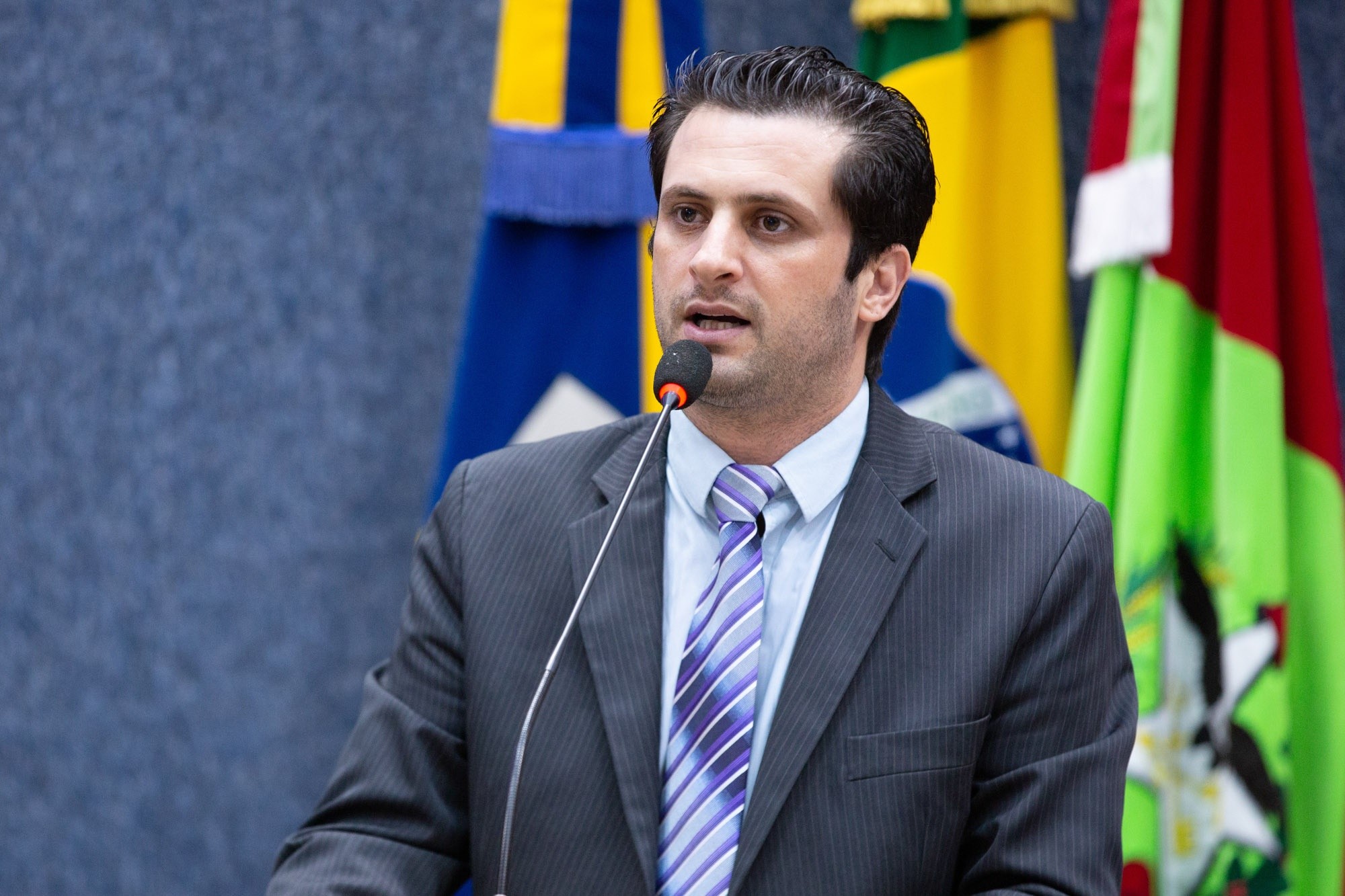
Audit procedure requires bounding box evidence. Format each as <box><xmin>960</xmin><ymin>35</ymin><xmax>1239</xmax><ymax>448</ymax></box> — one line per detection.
<box><xmin>498</xmin><ymin>338</ymin><xmax>714</xmax><ymax>896</ymax></box>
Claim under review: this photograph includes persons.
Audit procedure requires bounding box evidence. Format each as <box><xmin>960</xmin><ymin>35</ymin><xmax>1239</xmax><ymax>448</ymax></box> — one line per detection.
<box><xmin>265</xmin><ymin>47</ymin><xmax>1138</xmax><ymax>896</ymax></box>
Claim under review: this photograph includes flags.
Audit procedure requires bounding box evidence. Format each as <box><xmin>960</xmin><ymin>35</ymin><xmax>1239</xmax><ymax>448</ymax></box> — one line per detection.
<box><xmin>422</xmin><ymin>2</ymin><xmax>1345</xmax><ymax>896</ymax></box>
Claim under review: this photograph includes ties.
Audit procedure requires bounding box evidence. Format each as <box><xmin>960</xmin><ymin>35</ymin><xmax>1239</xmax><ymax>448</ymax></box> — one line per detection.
<box><xmin>655</xmin><ymin>463</ymin><xmax>787</xmax><ymax>896</ymax></box>
